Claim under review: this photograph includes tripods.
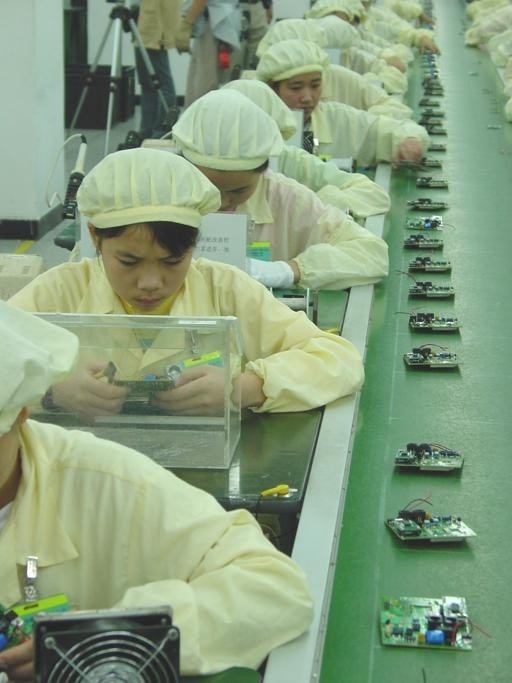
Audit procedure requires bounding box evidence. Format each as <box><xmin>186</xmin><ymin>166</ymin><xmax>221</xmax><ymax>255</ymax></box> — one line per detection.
<box><xmin>64</xmin><ymin>0</ymin><xmax>170</xmax><ymax>159</ymax></box>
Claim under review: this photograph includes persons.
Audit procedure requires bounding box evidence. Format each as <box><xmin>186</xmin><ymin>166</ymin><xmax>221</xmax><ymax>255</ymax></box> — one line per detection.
<box><xmin>0</xmin><ymin>299</ymin><xmax>315</xmax><ymax>683</ymax></box>
<box><xmin>465</xmin><ymin>1</ymin><xmax>512</xmax><ymax>124</ymax></box>
<box><xmin>4</xmin><ymin>147</ymin><xmax>365</xmax><ymax>424</ymax></box>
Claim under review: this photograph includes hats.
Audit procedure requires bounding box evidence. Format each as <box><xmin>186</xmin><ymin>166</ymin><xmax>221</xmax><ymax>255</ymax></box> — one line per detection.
<box><xmin>256</xmin><ymin>41</ymin><xmax>329</xmax><ymax>83</ymax></box>
<box><xmin>215</xmin><ymin>82</ymin><xmax>297</xmax><ymax>139</ymax></box>
<box><xmin>167</xmin><ymin>90</ymin><xmax>284</xmax><ymax>175</ymax></box>
<box><xmin>0</xmin><ymin>298</ymin><xmax>82</xmax><ymax>438</ymax></box>
<box><xmin>76</xmin><ymin>145</ymin><xmax>222</xmax><ymax>230</ymax></box>
<box><xmin>305</xmin><ymin>0</ymin><xmax>367</xmax><ymax>24</ymax></box>
<box><xmin>257</xmin><ymin>16</ymin><xmax>327</xmax><ymax>52</ymax></box>
<box><xmin>309</xmin><ymin>20</ymin><xmax>357</xmax><ymax>45</ymax></box>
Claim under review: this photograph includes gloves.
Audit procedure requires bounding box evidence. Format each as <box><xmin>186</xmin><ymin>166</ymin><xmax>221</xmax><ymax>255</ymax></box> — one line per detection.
<box><xmin>240</xmin><ymin>258</ymin><xmax>295</xmax><ymax>289</ymax></box>
